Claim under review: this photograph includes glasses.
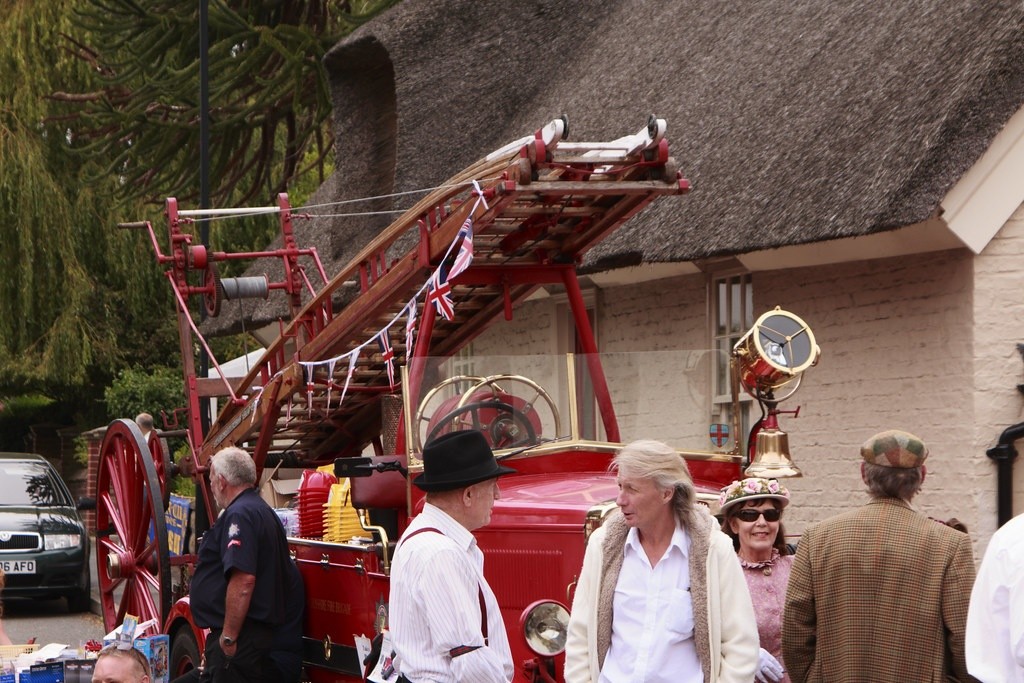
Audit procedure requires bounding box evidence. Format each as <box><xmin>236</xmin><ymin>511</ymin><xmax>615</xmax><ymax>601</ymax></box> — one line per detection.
<box><xmin>734</xmin><ymin>508</ymin><xmax>783</xmax><ymax>522</ymax></box>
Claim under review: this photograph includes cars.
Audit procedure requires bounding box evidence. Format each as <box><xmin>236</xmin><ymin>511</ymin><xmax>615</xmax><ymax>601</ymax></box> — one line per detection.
<box><xmin>0</xmin><ymin>453</ymin><xmax>96</xmax><ymax>613</ymax></box>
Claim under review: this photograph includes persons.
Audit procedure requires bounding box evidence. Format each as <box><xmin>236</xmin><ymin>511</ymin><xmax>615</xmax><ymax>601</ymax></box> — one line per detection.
<box><xmin>562</xmin><ymin>441</ymin><xmax>758</xmax><ymax>683</ymax></box>
<box><xmin>92</xmin><ymin>646</ymin><xmax>151</xmax><ymax>683</ymax></box>
<box><xmin>717</xmin><ymin>477</ymin><xmax>796</xmax><ymax>682</ymax></box>
<box><xmin>134</xmin><ymin>410</ymin><xmax>170</xmax><ymax>512</ymax></box>
<box><xmin>782</xmin><ymin>431</ymin><xmax>975</xmax><ymax>682</ymax></box>
<box><xmin>964</xmin><ymin>507</ymin><xmax>1024</xmax><ymax>683</ymax></box>
<box><xmin>188</xmin><ymin>448</ymin><xmax>305</xmax><ymax>683</ymax></box>
<box><xmin>388</xmin><ymin>430</ymin><xmax>515</xmax><ymax>683</ymax></box>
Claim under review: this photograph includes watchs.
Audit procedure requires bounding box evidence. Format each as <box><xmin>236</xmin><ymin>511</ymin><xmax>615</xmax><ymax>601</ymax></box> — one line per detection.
<box><xmin>220</xmin><ymin>632</ymin><xmax>237</xmax><ymax>646</ymax></box>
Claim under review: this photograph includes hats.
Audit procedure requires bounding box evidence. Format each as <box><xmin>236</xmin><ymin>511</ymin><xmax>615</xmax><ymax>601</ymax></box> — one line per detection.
<box><xmin>860</xmin><ymin>429</ymin><xmax>930</xmax><ymax>469</ymax></box>
<box><xmin>412</xmin><ymin>429</ymin><xmax>519</xmax><ymax>493</ymax></box>
<box><xmin>718</xmin><ymin>477</ymin><xmax>791</xmax><ymax>511</ymax></box>
